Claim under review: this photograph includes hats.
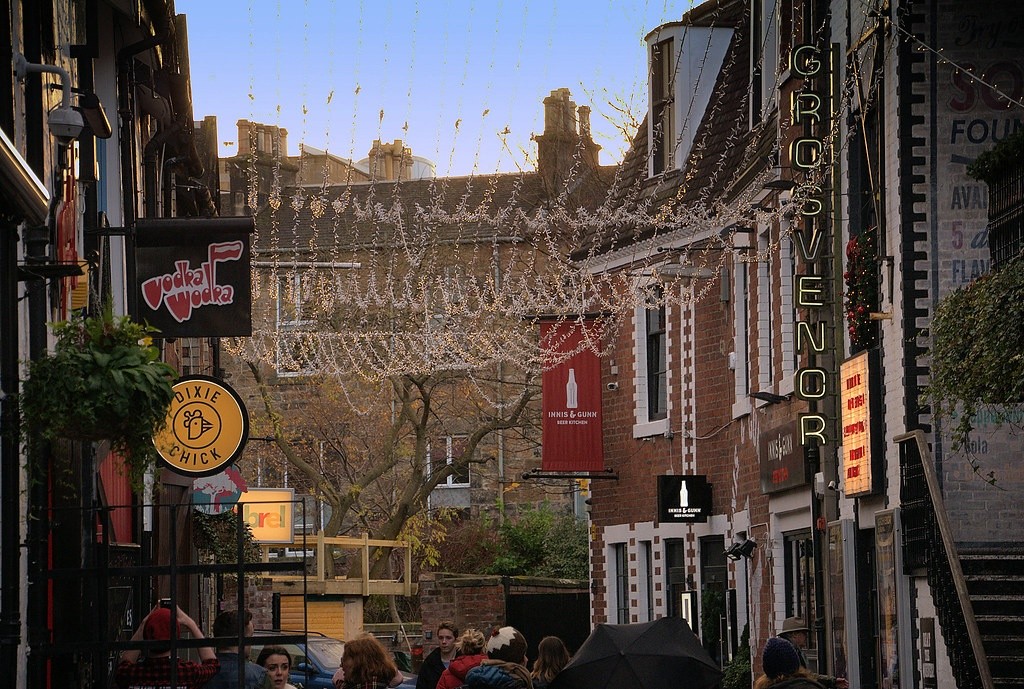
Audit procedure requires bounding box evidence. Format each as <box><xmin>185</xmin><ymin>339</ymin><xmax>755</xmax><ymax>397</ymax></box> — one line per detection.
<box><xmin>142</xmin><ymin>608</ymin><xmax>180</xmax><ymax>642</ymax></box>
<box><xmin>762</xmin><ymin>638</ymin><xmax>800</xmax><ymax>680</ymax></box>
<box><xmin>777</xmin><ymin>616</ymin><xmax>813</xmax><ymax>636</ymax></box>
<box><xmin>486</xmin><ymin>626</ymin><xmax>527</xmax><ymax>663</ymax></box>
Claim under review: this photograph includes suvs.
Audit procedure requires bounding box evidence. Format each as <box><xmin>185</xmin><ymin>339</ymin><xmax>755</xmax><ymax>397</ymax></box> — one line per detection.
<box><xmin>246</xmin><ymin>626</ymin><xmax>420</xmax><ymax>689</ymax></box>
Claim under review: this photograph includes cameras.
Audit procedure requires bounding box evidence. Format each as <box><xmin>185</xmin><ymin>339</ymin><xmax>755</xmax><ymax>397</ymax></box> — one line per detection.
<box><xmin>160</xmin><ymin>598</ymin><xmax>171</xmax><ymax>610</ymax></box>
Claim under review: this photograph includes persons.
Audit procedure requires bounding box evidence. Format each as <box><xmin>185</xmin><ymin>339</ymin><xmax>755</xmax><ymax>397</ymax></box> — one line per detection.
<box><xmin>332</xmin><ymin>630</ymin><xmax>403</xmax><ymax>689</ymax></box>
<box><xmin>753</xmin><ymin>616</ymin><xmax>849</xmax><ymax>689</ymax></box>
<box><xmin>415</xmin><ymin>620</ymin><xmax>572</xmax><ymax>689</ymax></box>
<box><xmin>113</xmin><ymin>599</ymin><xmax>298</xmax><ymax>689</ymax></box>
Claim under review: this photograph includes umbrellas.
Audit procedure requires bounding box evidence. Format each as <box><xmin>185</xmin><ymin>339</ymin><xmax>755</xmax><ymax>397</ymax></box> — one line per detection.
<box><xmin>546</xmin><ymin>616</ymin><xmax>726</xmax><ymax>689</ymax></box>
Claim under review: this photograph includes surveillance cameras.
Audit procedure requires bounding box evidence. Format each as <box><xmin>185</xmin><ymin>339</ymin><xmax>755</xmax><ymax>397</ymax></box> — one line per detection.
<box><xmin>827</xmin><ymin>480</ymin><xmax>835</xmax><ymax>490</ymax></box>
<box><xmin>47</xmin><ymin>109</ymin><xmax>83</xmax><ymax>145</ymax></box>
